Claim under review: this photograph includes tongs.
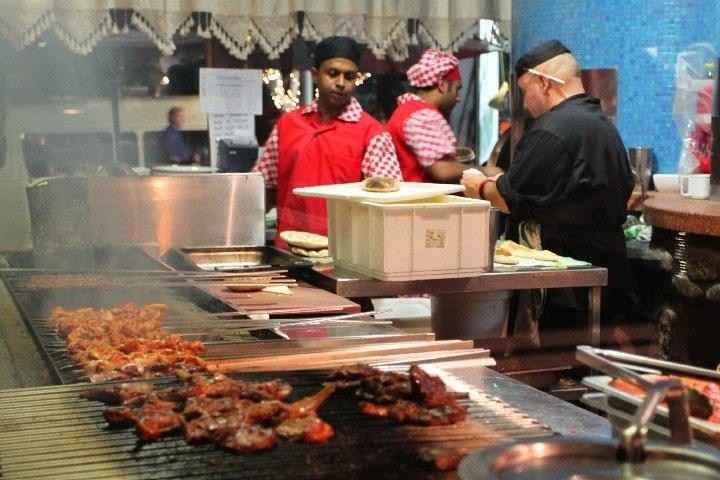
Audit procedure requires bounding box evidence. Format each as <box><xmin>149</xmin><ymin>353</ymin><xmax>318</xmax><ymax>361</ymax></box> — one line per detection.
<box><xmin>573</xmin><ymin>344</ymin><xmax>720</xmax><ymax>421</ymax></box>
<box><xmin>614</xmin><ymin>380</ymin><xmax>694</xmax><ymax>463</ymax></box>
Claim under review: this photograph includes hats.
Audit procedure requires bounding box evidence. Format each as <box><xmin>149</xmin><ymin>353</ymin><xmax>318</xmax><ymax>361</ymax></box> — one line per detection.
<box><xmin>515</xmin><ymin>40</ymin><xmax>571</xmax><ymax>80</ymax></box>
<box><xmin>406</xmin><ymin>49</ymin><xmax>461</xmax><ymax>86</ymax></box>
<box><xmin>314</xmin><ymin>36</ymin><xmax>363</xmax><ymax>68</ymax></box>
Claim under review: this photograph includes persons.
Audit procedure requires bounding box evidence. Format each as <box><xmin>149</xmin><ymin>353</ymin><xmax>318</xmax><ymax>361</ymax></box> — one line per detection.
<box><xmin>460</xmin><ymin>39</ymin><xmax>635</xmax><ymax>333</ymax></box>
<box><xmin>250</xmin><ymin>35</ymin><xmax>403</xmax><ymax>250</ymax></box>
<box><xmin>159</xmin><ymin>106</ymin><xmax>201</xmax><ymax>167</ymax></box>
<box><xmin>385</xmin><ymin>48</ymin><xmax>505</xmax><ymax>183</ymax></box>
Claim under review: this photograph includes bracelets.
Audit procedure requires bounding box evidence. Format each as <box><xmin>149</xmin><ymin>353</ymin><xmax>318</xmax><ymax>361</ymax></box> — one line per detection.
<box><xmin>479</xmin><ymin>178</ymin><xmax>496</xmax><ymax>200</ymax></box>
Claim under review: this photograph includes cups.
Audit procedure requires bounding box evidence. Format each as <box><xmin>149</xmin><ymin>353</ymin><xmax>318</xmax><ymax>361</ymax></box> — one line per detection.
<box><xmin>680</xmin><ymin>173</ymin><xmax>712</xmax><ymax>201</ymax></box>
<box><xmin>626</xmin><ymin>146</ymin><xmax>653</xmax><ymax>188</ymax></box>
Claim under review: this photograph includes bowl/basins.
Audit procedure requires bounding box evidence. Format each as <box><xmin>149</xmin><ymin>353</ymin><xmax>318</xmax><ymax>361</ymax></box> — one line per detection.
<box><xmin>651</xmin><ymin>173</ymin><xmax>680</xmax><ymax>194</ymax></box>
<box><xmin>458</xmin><ymin>434</ymin><xmax>720</xmax><ymax>480</ymax></box>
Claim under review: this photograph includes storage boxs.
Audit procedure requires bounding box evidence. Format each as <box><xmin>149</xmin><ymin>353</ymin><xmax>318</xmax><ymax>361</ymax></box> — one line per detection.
<box><xmin>289</xmin><ymin>176</ymin><xmax>493</xmax><ymax>284</ymax></box>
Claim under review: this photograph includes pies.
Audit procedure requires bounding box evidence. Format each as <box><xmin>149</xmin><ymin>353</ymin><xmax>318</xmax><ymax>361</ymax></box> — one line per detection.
<box><xmin>280</xmin><ymin>230</ymin><xmax>329</xmax><ymax>259</ymax></box>
<box><xmin>494</xmin><ymin>239</ymin><xmax>561</xmax><ymax>264</ymax></box>
<box><xmin>362</xmin><ymin>175</ymin><xmax>400</xmax><ymax>190</ymax></box>
